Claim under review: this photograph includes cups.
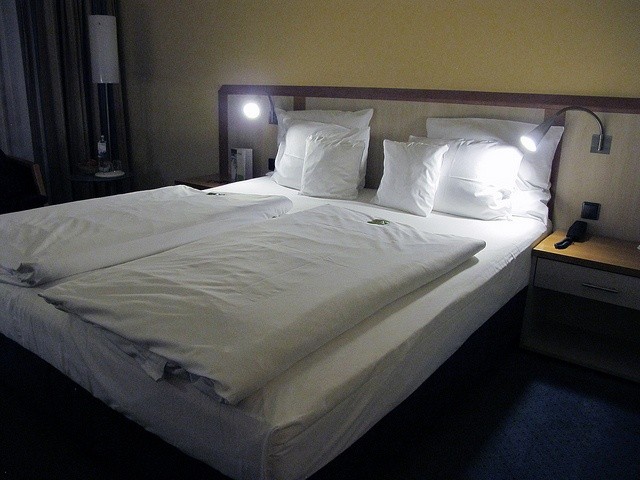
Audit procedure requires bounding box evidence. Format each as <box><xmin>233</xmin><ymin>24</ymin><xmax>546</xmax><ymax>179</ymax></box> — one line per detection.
<box><xmin>114</xmin><ymin>161</ymin><xmax>121</xmax><ymax>172</ymax></box>
<box><xmin>103</xmin><ymin>160</ymin><xmax>112</xmax><ymax>174</ymax></box>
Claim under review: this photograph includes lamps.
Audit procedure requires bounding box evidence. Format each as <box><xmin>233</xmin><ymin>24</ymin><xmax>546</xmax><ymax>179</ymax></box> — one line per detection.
<box><xmin>87</xmin><ymin>14</ymin><xmax>125</xmax><ymax>179</ymax></box>
<box><xmin>519</xmin><ymin>105</ymin><xmax>612</xmax><ymax>155</ymax></box>
<box><xmin>243</xmin><ymin>90</ymin><xmax>277</xmax><ymax>176</ymax></box>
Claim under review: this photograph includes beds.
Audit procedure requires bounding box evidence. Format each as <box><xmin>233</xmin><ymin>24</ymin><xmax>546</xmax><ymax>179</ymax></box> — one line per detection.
<box><xmin>0</xmin><ymin>84</ymin><xmax>640</xmax><ymax>478</ymax></box>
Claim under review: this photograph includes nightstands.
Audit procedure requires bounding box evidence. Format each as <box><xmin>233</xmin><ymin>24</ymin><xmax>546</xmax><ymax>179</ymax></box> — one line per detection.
<box><xmin>519</xmin><ymin>228</ymin><xmax>640</xmax><ymax>383</ymax></box>
<box><xmin>175</xmin><ymin>174</ymin><xmax>236</xmax><ymax>190</ymax></box>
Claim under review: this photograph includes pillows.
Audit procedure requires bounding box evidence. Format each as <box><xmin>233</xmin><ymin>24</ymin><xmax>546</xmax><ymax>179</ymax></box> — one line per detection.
<box><xmin>298</xmin><ymin>136</ymin><xmax>365</xmax><ymax>200</ymax></box>
<box><xmin>426</xmin><ymin>118</ymin><xmax>565</xmax><ymax>226</ymax></box>
<box><xmin>272</xmin><ymin>105</ymin><xmax>374</xmax><ymax>174</ymax></box>
<box><xmin>408</xmin><ymin>134</ymin><xmax>523</xmax><ymax>220</ymax></box>
<box><xmin>370</xmin><ymin>139</ymin><xmax>449</xmax><ymax>217</ymax></box>
<box><xmin>271</xmin><ymin>116</ymin><xmax>370</xmax><ymax>193</ymax></box>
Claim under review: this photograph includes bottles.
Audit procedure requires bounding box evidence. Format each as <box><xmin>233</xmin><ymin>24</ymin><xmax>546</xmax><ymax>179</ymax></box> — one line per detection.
<box><xmin>97</xmin><ymin>134</ymin><xmax>110</xmax><ymax>170</ymax></box>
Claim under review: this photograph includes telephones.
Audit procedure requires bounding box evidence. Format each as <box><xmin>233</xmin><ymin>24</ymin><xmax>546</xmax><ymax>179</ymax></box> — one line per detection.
<box><xmin>554</xmin><ymin>220</ymin><xmax>587</xmax><ymax>249</ymax></box>
